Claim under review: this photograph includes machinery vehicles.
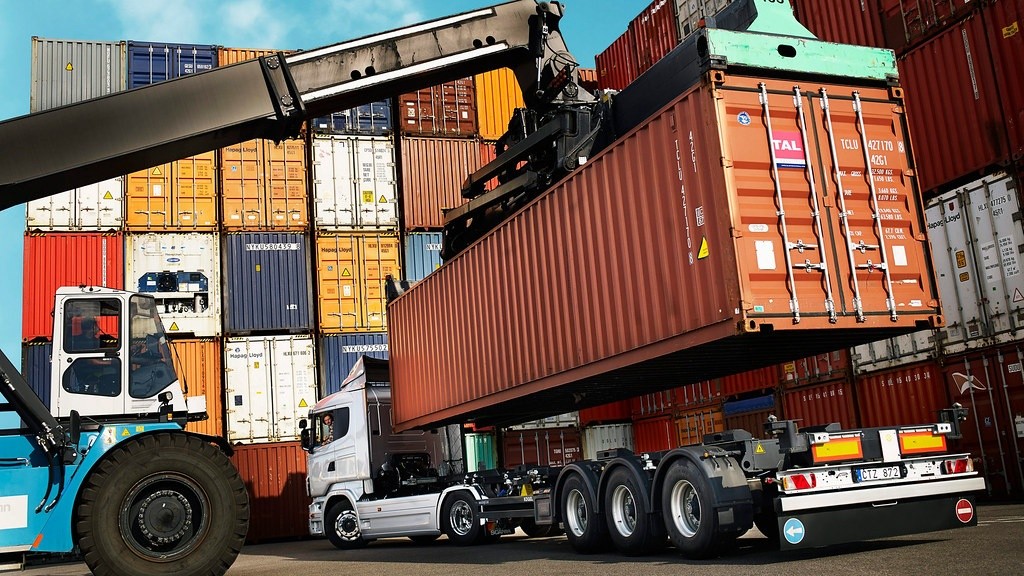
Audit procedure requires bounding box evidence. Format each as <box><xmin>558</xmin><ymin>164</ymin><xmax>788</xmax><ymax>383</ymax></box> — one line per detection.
<box><xmin>1</xmin><ymin>0</ymin><xmax>617</xmax><ymax>576</ymax></box>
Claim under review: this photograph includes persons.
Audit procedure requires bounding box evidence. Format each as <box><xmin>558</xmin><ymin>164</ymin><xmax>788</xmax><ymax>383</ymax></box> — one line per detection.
<box><xmin>321</xmin><ymin>412</ymin><xmax>335</xmax><ymax>445</ymax></box>
<box><xmin>74</xmin><ymin>316</ymin><xmax>121</xmax><ymax>396</ymax></box>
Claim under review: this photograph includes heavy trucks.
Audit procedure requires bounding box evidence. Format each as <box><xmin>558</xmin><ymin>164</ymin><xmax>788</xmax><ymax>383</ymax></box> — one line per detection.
<box><xmin>298</xmin><ymin>354</ymin><xmax>986</xmax><ymax>561</ymax></box>
